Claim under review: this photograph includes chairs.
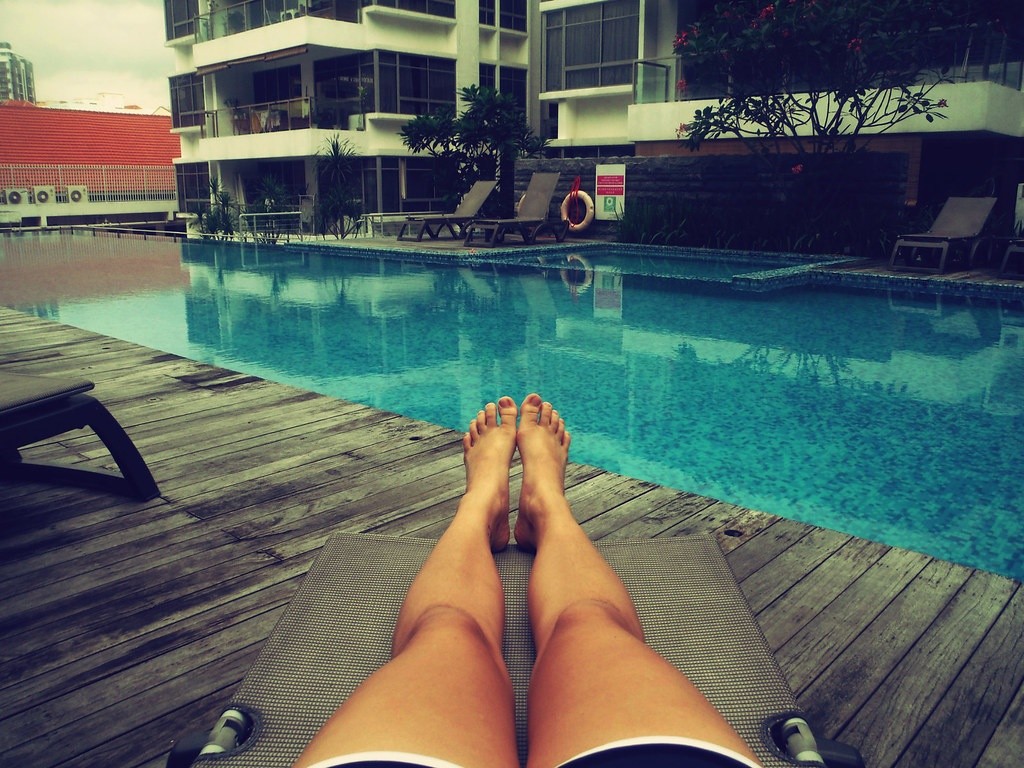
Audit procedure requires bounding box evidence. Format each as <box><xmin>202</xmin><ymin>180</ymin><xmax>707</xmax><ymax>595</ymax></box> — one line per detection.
<box><xmin>463</xmin><ymin>171</ymin><xmax>570</xmax><ymax>247</ymax></box>
<box><xmin>887</xmin><ymin>195</ymin><xmax>998</xmax><ymax>276</ymax></box>
<box><xmin>396</xmin><ymin>180</ymin><xmax>499</xmax><ymax>243</ymax></box>
<box><xmin>1</xmin><ymin>369</ymin><xmax>161</xmax><ymax>500</ymax></box>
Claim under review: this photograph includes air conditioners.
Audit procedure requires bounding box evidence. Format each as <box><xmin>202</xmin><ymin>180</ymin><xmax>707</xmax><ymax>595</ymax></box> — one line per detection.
<box><xmin>33</xmin><ymin>185</ymin><xmax>56</xmax><ymax>204</ymax></box>
<box><xmin>38</xmin><ymin>230</ymin><xmax>61</xmax><ymax>246</ymax></box>
<box><xmin>72</xmin><ymin>229</ymin><xmax>93</xmax><ymax>244</ymax></box>
<box><xmin>4</xmin><ymin>188</ymin><xmax>29</xmax><ymax>205</ymax></box>
<box><xmin>9</xmin><ymin>232</ymin><xmax>33</xmax><ymax>247</ymax></box>
<box><xmin>66</xmin><ymin>184</ymin><xmax>88</xmax><ymax>203</ymax></box>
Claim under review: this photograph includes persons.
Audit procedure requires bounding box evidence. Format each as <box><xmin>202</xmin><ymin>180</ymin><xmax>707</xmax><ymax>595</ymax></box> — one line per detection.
<box><xmin>294</xmin><ymin>394</ymin><xmax>763</xmax><ymax>768</ymax></box>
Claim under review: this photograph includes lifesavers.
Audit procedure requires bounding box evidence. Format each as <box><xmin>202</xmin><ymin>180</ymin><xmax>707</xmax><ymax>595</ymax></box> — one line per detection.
<box><xmin>560</xmin><ymin>190</ymin><xmax>594</xmax><ymax>233</ymax></box>
<box><xmin>559</xmin><ymin>252</ymin><xmax>594</xmax><ymax>296</ymax></box>
